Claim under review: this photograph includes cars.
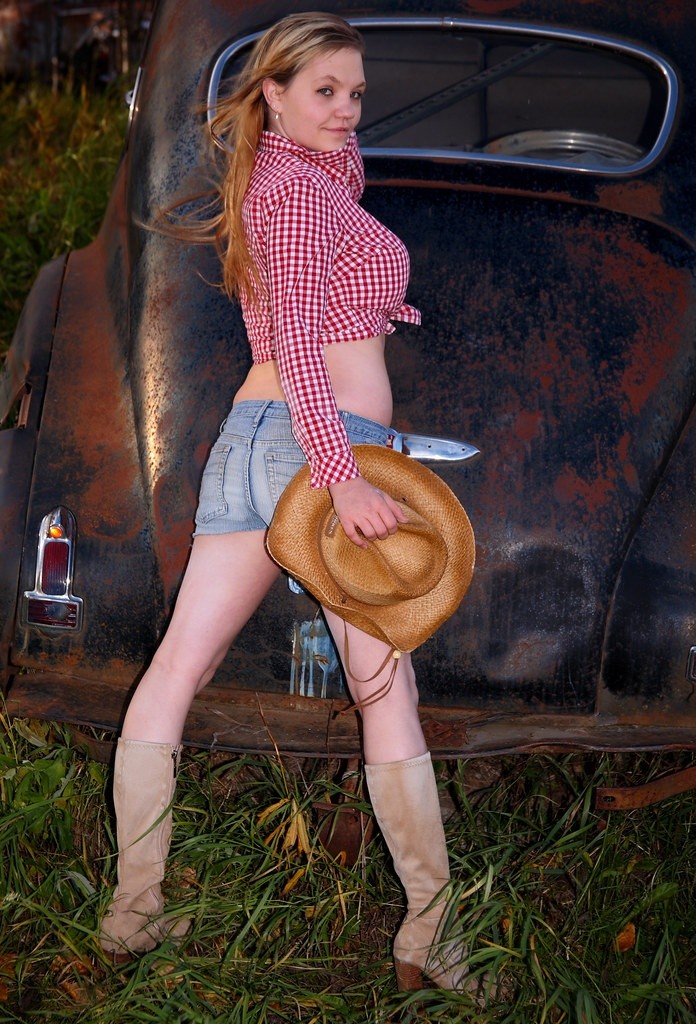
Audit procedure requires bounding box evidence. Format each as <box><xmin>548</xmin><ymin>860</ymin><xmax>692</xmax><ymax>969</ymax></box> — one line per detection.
<box><xmin>0</xmin><ymin>2</ymin><xmax>696</xmax><ymax>819</ymax></box>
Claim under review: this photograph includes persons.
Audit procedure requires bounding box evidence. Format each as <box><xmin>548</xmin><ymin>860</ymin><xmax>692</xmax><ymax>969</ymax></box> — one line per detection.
<box><xmin>96</xmin><ymin>12</ymin><xmax>513</xmax><ymax>1002</ymax></box>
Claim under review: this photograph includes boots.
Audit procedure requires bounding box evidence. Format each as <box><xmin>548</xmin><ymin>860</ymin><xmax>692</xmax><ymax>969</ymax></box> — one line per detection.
<box><xmin>364</xmin><ymin>749</ymin><xmax>511</xmax><ymax>1005</ymax></box>
<box><xmin>101</xmin><ymin>738</ymin><xmax>192</xmax><ymax>964</ymax></box>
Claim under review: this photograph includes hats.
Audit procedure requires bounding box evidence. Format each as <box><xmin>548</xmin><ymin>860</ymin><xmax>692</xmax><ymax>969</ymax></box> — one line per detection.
<box><xmin>267</xmin><ymin>443</ymin><xmax>478</xmax><ymax>654</ymax></box>
